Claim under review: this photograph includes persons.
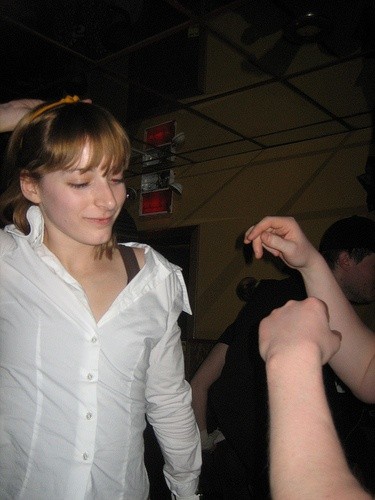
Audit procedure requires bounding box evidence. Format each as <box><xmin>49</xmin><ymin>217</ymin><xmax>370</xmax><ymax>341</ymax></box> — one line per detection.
<box><xmin>0</xmin><ymin>94</ymin><xmax>206</xmax><ymax>500</ymax></box>
<box><xmin>238</xmin><ymin>214</ymin><xmax>375</xmax><ymax>500</ymax></box>
<box><xmin>193</xmin><ymin>218</ymin><xmax>375</xmax><ymax>499</ymax></box>
